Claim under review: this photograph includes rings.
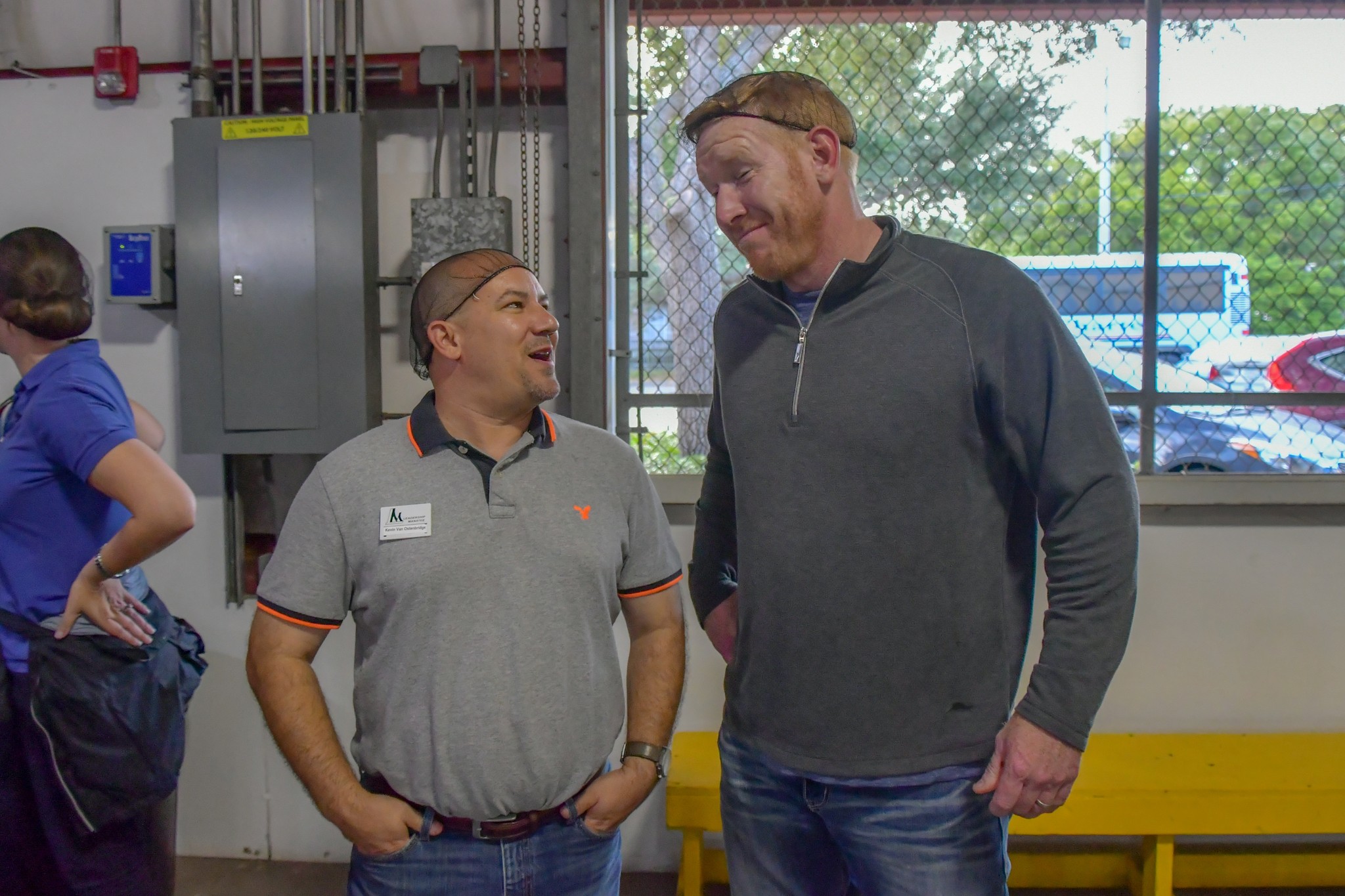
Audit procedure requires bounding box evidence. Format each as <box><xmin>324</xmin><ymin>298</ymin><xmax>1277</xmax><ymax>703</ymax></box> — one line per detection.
<box><xmin>1036</xmin><ymin>799</ymin><xmax>1055</xmax><ymax>809</ymax></box>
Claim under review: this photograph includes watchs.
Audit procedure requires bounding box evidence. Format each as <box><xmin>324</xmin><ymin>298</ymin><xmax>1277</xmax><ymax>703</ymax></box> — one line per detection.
<box><xmin>619</xmin><ymin>741</ymin><xmax>672</xmax><ymax>781</ymax></box>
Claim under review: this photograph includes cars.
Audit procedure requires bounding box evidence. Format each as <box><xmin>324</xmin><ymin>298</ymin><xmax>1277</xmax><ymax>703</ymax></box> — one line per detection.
<box><xmin>995</xmin><ymin>254</ymin><xmax>1345</xmax><ymax>475</ymax></box>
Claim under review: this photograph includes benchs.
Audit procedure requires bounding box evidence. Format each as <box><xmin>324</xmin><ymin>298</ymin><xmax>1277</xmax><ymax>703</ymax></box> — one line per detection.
<box><xmin>661</xmin><ymin>726</ymin><xmax>1344</xmax><ymax>896</ymax></box>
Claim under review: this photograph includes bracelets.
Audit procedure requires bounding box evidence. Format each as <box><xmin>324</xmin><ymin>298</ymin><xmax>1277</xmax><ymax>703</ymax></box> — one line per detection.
<box><xmin>95</xmin><ymin>543</ymin><xmax>132</xmax><ymax>580</ymax></box>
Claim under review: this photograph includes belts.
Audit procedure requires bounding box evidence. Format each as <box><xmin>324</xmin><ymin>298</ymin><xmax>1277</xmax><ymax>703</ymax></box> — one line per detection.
<box><xmin>358</xmin><ymin>759</ymin><xmax>606</xmax><ymax>842</ymax></box>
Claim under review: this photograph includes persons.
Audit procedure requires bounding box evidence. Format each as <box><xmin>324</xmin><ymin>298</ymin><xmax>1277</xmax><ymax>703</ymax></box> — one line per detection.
<box><xmin>243</xmin><ymin>246</ymin><xmax>692</xmax><ymax>896</ymax></box>
<box><xmin>1</xmin><ymin>227</ymin><xmax>211</xmax><ymax>892</ymax></box>
<box><xmin>682</xmin><ymin>70</ymin><xmax>1144</xmax><ymax>894</ymax></box>
